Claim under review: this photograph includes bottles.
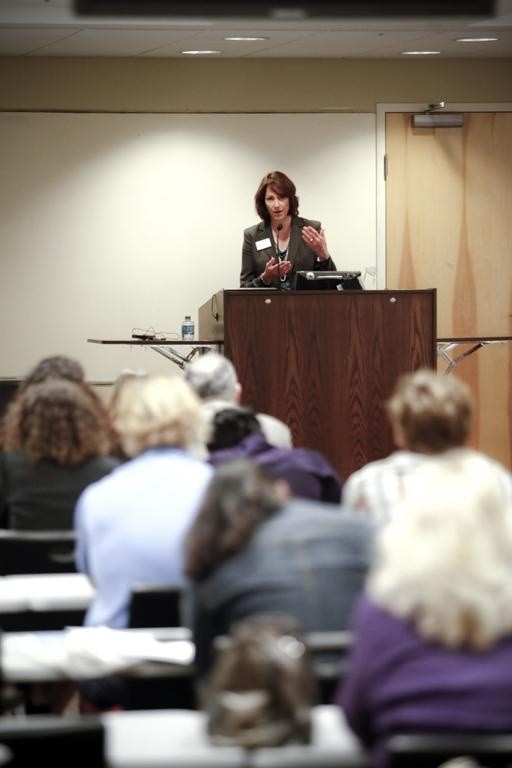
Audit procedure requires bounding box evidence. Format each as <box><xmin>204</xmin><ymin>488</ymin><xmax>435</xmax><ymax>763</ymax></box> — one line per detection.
<box><xmin>180</xmin><ymin>315</ymin><xmax>196</xmax><ymax>341</ymax></box>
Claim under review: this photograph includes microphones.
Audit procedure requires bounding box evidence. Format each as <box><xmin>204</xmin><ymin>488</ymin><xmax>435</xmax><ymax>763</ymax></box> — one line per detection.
<box><xmin>275</xmin><ymin>222</ymin><xmax>283</xmax><ymax>289</ymax></box>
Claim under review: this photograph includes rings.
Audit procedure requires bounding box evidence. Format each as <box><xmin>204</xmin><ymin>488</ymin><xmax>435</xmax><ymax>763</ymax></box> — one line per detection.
<box><xmin>310</xmin><ymin>238</ymin><xmax>314</xmax><ymax>243</ymax></box>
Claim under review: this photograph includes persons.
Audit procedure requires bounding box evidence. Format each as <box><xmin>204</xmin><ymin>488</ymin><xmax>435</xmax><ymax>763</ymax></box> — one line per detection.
<box><xmin>1</xmin><ymin>353</ymin><xmax>512</xmax><ymax>643</ymax></box>
<box><xmin>239</xmin><ymin>170</ymin><xmax>338</xmax><ymax>288</ymax></box>
<box><xmin>333</xmin><ymin>467</ymin><xmax>511</xmax><ymax>755</ymax></box>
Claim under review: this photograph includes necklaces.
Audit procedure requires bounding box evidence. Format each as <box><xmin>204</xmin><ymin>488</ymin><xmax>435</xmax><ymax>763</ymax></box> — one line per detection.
<box><xmin>276</xmin><ymin>245</ymin><xmax>288</xmax><ymax>281</ymax></box>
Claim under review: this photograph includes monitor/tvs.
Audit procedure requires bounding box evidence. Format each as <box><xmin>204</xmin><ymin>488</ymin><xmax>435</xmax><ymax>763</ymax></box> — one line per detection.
<box><xmin>295</xmin><ymin>266</ymin><xmax>361</xmax><ymax>290</ymax></box>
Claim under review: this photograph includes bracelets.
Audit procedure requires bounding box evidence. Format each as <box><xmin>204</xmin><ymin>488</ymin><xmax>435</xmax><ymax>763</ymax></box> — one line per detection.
<box><xmin>260</xmin><ymin>273</ymin><xmax>272</xmax><ymax>286</ymax></box>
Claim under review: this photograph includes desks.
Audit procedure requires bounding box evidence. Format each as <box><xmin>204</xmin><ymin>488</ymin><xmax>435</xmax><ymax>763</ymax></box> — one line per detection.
<box><xmin>1</xmin><ymin>527</ymin><xmax>363</xmax><ymax>768</ymax></box>
